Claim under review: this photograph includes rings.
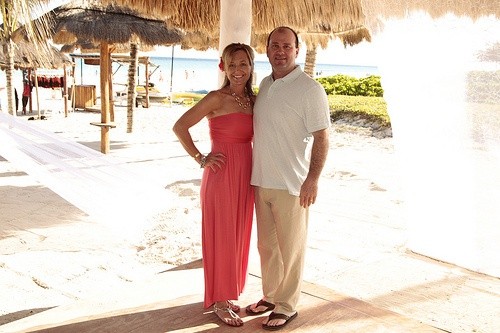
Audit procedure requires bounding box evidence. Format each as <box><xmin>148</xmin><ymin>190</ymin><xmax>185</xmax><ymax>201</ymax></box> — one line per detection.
<box><xmin>309</xmin><ymin>200</ymin><xmax>313</xmax><ymax>202</ymax></box>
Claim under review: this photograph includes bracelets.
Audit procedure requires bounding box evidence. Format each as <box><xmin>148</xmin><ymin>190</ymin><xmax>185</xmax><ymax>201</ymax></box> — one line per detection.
<box><xmin>194</xmin><ymin>152</ymin><xmax>202</xmax><ymax>158</ymax></box>
<box><xmin>198</xmin><ymin>156</ymin><xmax>208</xmax><ymax>165</ymax></box>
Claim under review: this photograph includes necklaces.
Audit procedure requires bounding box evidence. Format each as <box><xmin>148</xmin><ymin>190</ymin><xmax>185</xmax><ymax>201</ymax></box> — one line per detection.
<box><xmin>231</xmin><ymin>91</ymin><xmax>251</xmax><ymax>110</ymax></box>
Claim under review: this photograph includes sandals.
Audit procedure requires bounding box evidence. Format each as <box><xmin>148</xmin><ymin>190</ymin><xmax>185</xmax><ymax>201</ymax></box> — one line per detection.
<box><xmin>227</xmin><ymin>301</ymin><xmax>240</xmax><ymax>313</ymax></box>
<box><xmin>214</xmin><ymin>304</ymin><xmax>243</xmax><ymax>327</ymax></box>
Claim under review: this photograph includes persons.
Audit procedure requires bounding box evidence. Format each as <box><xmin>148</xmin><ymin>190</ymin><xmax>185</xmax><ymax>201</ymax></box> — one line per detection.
<box><xmin>21</xmin><ymin>79</ymin><xmax>30</xmax><ymax>116</ymax></box>
<box><xmin>173</xmin><ymin>42</ymin><xmax>257</xmax><ymax>327</ymax></box>
<box><xmin>245</xmin><ymin>27</ymin><xmax>331</xmax><ymax>331</ymax></box>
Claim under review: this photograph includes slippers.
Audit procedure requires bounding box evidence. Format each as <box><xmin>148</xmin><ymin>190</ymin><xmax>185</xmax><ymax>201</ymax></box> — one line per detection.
<box><xmin>262</xmin><ymin>312</ymin><xmax>298</xmax><ymax>330</ymax></box>
<box><xmin>246</xmin><ymin>299</ymin><xmax>275</xmax><ymax>314</ymax></box>
